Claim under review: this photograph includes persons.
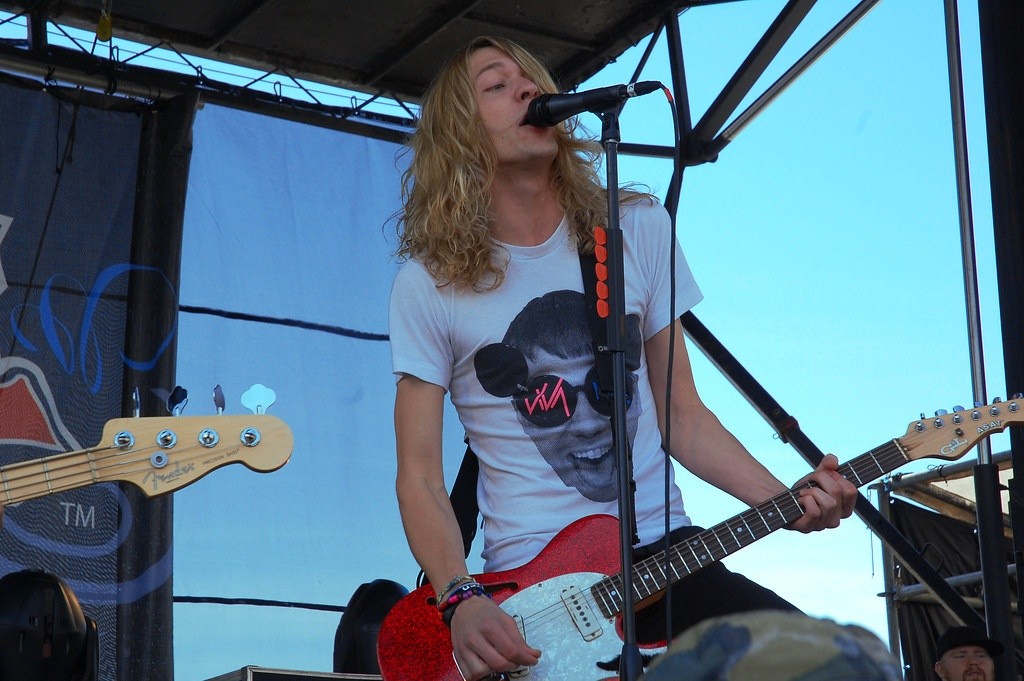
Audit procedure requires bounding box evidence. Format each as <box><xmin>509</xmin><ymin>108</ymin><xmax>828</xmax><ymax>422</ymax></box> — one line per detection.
<box><xmin>933</xmin><ymin>626</ymin><xmax>1006</xmax><ymax>681</ymax></box>
<box><xmin>387</xmin><ymin>37</ymin><xmax>858</xmax><ymax>681</ymax></box>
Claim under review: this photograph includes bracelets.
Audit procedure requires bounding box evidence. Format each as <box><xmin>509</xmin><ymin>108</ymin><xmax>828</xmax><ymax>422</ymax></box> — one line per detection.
<box><xmin>436</xmin><ymin>574</ymin><xmax>486</xmax><ymax>626</ymax></box>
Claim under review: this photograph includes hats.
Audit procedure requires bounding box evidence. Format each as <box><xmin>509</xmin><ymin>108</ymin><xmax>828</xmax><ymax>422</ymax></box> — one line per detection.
<box><xmin>934</xmin><ymin>625</ymin><xmax>1003</xmax><ymax>661</ymax></box>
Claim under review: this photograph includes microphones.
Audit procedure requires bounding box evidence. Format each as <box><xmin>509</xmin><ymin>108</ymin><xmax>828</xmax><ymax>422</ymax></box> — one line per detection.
<box><xmin>528</xmin><ymin>81</ymin><xmax>661</xmax><ymax>128</ymax></box>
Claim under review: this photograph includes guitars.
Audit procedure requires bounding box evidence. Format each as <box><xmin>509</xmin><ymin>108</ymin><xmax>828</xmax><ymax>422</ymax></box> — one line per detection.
<box><xmin>0</xmin><ymin>382</ymin><xmax>303</xmax><ymax>520</ymax></box>
<box><xmin>370</xmin><ymin>389</ymin><xmax>1024</xmax><ymax>681</ymax></box>
